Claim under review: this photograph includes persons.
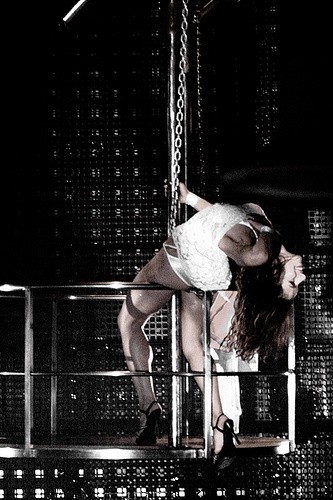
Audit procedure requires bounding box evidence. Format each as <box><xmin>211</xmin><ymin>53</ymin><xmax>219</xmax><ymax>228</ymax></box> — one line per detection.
<box><xmin>116</xmin><ymin>177</ymin><xmax>307</xmax><ymax>473</ymax></box>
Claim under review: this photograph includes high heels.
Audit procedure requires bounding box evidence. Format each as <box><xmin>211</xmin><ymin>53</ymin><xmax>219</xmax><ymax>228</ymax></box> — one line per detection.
<box><xmin>212</xmin><ymin>412</ymin><xmax>241</xmax><ymax>470</ymax></box>
<box><xmin>136</xmin><ymin>399</ymin><xmax>163</xmax><ymax>444</ymax></box>
